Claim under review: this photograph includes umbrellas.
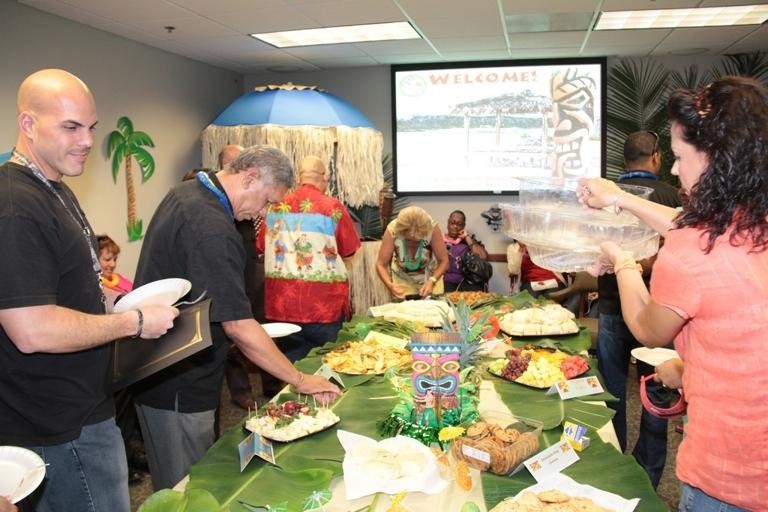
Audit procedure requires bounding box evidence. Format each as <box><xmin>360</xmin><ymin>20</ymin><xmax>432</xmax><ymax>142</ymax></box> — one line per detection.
<box><xmin>199</xmin><ymin>81</ymin><xmax>384</xmax><ymax>209</ymax></box>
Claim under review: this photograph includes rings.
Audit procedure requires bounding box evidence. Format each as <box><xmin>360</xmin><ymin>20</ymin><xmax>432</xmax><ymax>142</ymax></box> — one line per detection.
<box><xmin>662</xmin><ymin>384</ymin><xmax>667</xmax><ymax>388</ymax></box>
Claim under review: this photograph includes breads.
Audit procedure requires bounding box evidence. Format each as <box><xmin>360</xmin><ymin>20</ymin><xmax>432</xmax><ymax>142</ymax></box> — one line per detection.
<box><xmin>384</xmin><ymin>299</ymin><xmax>454</xmax><ymax>327</ymax></box>
<box><xmin>499</xmin><ymin>304</ymin><xmax>578</xmax><ymax>335</ymax></box>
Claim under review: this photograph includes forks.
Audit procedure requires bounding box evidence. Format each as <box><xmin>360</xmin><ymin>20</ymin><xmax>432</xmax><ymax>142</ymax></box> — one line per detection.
<box><xmin>173</xmin><ymin>290</ymin><xmax>209</xmax><ymax>310</ymax></box>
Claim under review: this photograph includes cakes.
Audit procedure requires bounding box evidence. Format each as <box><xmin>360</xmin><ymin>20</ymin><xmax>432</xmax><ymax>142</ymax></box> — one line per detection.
<box><xmin>245</xmin><ymin>399</ymin><xmax>337</xmax><ymax>440</ymax></box>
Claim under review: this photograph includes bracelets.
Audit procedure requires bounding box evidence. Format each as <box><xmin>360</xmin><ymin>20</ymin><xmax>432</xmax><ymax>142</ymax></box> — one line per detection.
<box><xmin>464</xmin><ymin>234</ymin><xmax>469</xmax><ymax>240</ymax></box>
<box><xmin>615</xmin><ymin>262</ymin><xmax>644</xmax><ymax>275</ymax></box>
<box><xmin>290</xmin><ymin>371</ymin><xmax>304</xmax><ymax>393</ymax></box>
<box><xmin>131</xmin><ymin>308</ymin><xmax>144</xmax><ymax>340</ymax></box>
<box><xmin>428</xmin><ymin>276</ymin><xmax>437</xmax><ymax>284</ymax></box>
<box><xmin>613</xmin><ymin>190</ymin><xmax>626</xmax><ymax>211</ymax></box>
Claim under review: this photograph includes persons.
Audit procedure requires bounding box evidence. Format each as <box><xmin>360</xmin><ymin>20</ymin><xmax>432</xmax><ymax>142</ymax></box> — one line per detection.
<box><xmin>595</xmin><ymin>129</ymin><xmax>685</xmax><ymax>495</ymax></box>
<box><xmin>375</xmin><ymin>206</ymin><xmax>450</xmax><ymax>301</ymax></box>
<box><xmin>507</xmin><ymin>235</ymin><xmax>581</xmax><ymax>316</ymax></box>
<box><xmin>440</xmin><ymin>210</ymin><xmax>489</xmax><ymax>291</ymax></box>
<box><xmin>652</xmin><ymin>357</ymin><xmax>686</xmax><ymax>391</ymax></box>
<box><xmin>574</xmin><ymin>74</ymin><xmax>768</xmax><ymax>512</ymax></box>
<box><xmin>254</xmin><ymin>155</ymin><xmax>362</xmax><ymax>367</ymax></box>
<box><xmin>215</xmin><ymin>146</ymin><xmax>285</xmax><ymax>411</ymax></box>
<box><xmin>95</xmin><ymin>233</ymin><xmax>149</xmax><ymax>486</ymax></box>
<box><xmin>1</xmin><ymin>68</ymin><xmax>180</xmax><ymax>512</ymax></box>
<box><xmin>127</xmin><ymin>144</ymin><xmax>296</xmax><ymax>492</ymax></box>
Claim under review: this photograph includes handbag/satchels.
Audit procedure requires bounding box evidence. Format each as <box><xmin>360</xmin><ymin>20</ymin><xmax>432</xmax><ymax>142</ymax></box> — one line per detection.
<box><xmin>461</xmin><ymin>240</ymin><xmax>493</xmax><ymax>286</ymax></box>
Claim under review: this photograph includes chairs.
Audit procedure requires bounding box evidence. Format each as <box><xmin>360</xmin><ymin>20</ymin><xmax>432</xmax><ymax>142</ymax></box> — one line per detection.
<box><xmin>565</xmin><ymin>289</ymin><xmax>600</xmax><ymax>357</ymax></box>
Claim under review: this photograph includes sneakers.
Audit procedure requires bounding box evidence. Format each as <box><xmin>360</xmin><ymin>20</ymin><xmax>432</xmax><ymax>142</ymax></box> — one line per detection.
<box><xmin>231</xmin><ymin>395</ymin><xmax>261</xmax><ymax>410</ymax></box>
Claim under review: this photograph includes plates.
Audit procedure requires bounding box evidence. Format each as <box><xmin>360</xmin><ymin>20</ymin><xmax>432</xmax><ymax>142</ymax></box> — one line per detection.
<box><xmin>112</xmin><ymin>273</ymin><xmax>191</xmax><ymax>316</ymax></box>
<box><xmin>0</xmin><ymin>442</ymin><xmax>45</xmax><ymax>505</ymax></box>
<box><xmin>260</xmin><ymin>322</ymin><xmax>303</xmax><ymax>339</ymax></box>
<box><xmin>319</xmin><ymin>355</ymin><xmax>386</xmax><ymax>376</ymax></box>
<box><xmin>626</xmin><ymin>344</ymin><xmax>681</xmax><ymax>366</ymax></box>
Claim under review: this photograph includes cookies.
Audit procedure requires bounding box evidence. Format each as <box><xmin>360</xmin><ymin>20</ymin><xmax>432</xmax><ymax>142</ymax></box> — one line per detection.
<box><xmin>489</xmin><ymin>488</ymin><xmax>610</xmax><ymax>512</ymax></box>
<box><xmin>456</xmin><ymin>421</ymin><xmax>541</xmax><ymax>475</ymax></box>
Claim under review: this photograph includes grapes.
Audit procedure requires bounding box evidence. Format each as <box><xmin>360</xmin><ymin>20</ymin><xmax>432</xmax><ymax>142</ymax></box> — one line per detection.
<box><xmin>501</xmin><ymin>354</ymin><xmax>530</xmax><ymax>381</ymax></box>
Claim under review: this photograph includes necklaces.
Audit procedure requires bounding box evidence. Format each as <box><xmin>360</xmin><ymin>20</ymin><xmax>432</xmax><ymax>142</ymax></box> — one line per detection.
<box><xmin>10</xmin><ymin>147</ymin><xmax>107</xmax><ymax>307</ymax></box>
